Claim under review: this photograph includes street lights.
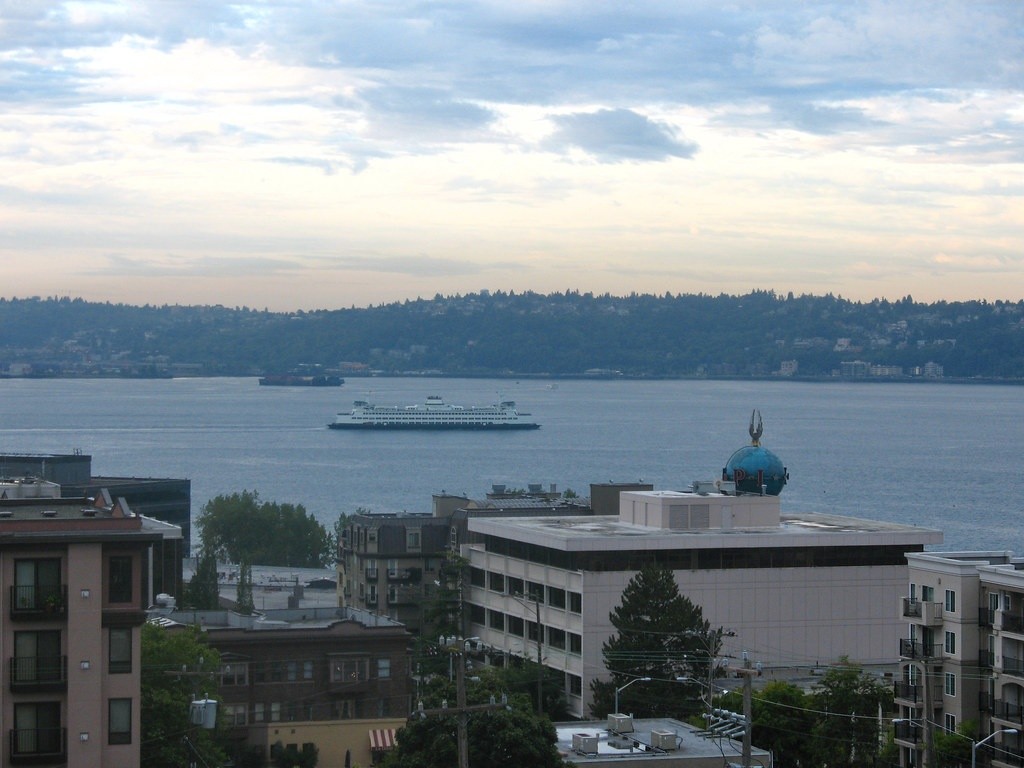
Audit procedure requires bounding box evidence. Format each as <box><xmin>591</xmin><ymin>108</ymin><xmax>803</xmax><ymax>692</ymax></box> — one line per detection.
<box><xmin>615</xmin><ymin>677</ymin><xmax>651</xmax><ymax>716</ymax></box>
<box><xmin>892</xmin><ymin>719</ymin><xmax>933</xmax><ymax>749</ymax></box>
<box><xmin>497</xmin><ymin>593</ymin><xmax>542</xmax><ymax>716</ymax></box>
<box><xmin>972</xmin><ymin>729</ymin><xmax>1019</xmax><ymax>768</ymax></box>
<box><xmin>676</xmin><ymin>675</ymin><xmax>712</xmax><ymax>731</ymax></box>
<box><xmin>463</xmin><ymin>636</ymin><xmax>480</xmax><ymax>659</ymax></box>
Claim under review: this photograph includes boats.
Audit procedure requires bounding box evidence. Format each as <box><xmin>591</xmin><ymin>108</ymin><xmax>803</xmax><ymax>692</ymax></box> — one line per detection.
<box><xmin>326</xmin><ymin>394</ymin><xmax>542</xmax><ymax>431</ymax></box>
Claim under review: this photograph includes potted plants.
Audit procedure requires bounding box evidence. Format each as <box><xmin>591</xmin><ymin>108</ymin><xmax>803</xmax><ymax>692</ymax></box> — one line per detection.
<box><xmin>48</xmin><ymin>590</ymin><xmax>61</xmax><ymax>610</ymax></box>
